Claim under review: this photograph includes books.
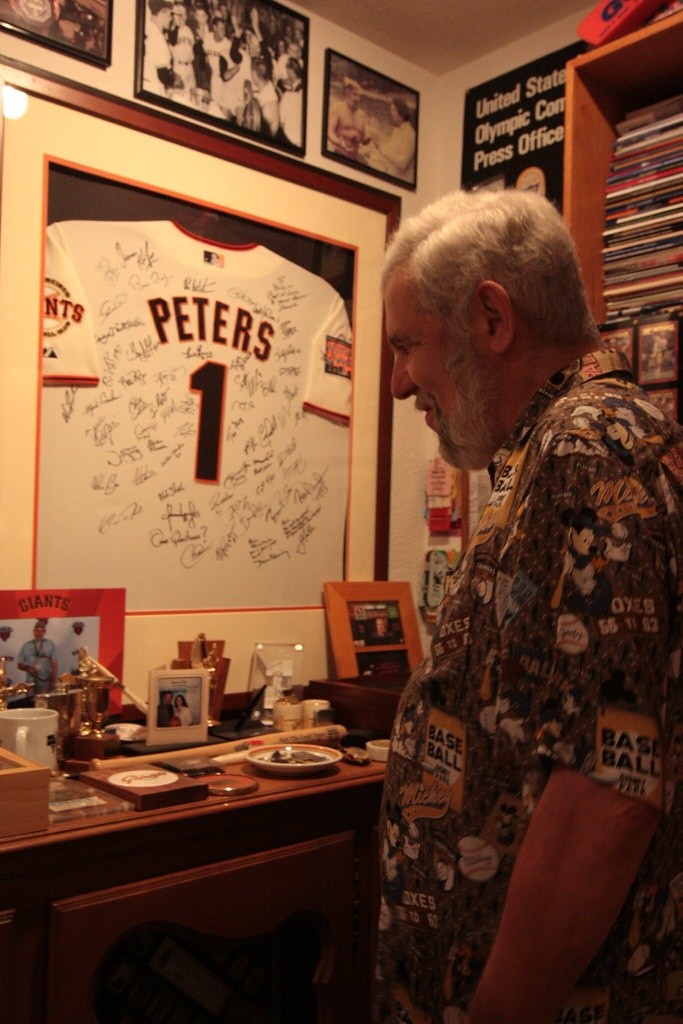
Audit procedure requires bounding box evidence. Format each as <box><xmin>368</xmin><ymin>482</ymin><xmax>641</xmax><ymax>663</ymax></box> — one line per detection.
<box><xmin>601</xmin><ymin>95</ymin><xmax>683</xmax><ymax>325</ymax></box>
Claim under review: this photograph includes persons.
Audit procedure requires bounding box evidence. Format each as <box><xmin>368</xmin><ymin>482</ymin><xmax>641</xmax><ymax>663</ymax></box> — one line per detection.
<box><xmin>156</xmin><ymin>691</ymin><xmax>193</xmax><ymax>728</ymax></box>
<box><xmin>649</xmin><ymin>328</ymin><xmax>668</xmax><ymax>380</ymax></box>
<box><xmin>606</xmin><ymin>339</ymin><xmax>622</xmax><ymax>350</ymax></box>
<box><xmin>328</xmin><ymin>78</ymin><xmax>416</xmax><ymax>176</ymax></box>
<box><xmin>0</xmin><ymin>0</ymin><xmax>106</xmax><ymax>57</ymax></box>
<box><xmin>17</xmin><ymin>623</ymin><xmax>58</xmax><ymax>691</ymax></box>
<box><xmin>145</xmin><ymin>0</ymin><xmax>303</xmax><ymax>149</ymax></box>
<box><xmin>372</xmin><ymin>192</ymin><xmax>683</xmax><ymax>1024</ymax></box>
<box><xmin>58</xmin><ymin>622</ymin><xmax>85</xmax><ymax>675</ymax></box>
<box><xmin>0</xmin><ymin>626</ymin><xmax>14</xmax><ymax>682</ymax></box>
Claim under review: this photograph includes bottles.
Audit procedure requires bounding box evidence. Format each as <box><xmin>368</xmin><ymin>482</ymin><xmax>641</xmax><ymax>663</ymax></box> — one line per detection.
<box><xmin>273</xmin><ymin>690</ymin><xmax>301</xmax><ymax>730</ymax></box>
<box><xmin>261</xmin><ymin>672</ymin><xmax>287</xmax><ymax>725</ymax></box>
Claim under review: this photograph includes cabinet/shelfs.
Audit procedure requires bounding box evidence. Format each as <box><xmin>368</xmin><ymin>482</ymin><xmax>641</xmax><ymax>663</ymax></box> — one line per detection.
<box><xmin>0</xmin><ymin>758</ymin><xmax>386</xmax><ymax>1024</ymax></box>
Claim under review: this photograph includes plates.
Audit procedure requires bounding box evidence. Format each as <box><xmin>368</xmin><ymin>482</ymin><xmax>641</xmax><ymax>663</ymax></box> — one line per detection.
<box><xmin>245</xmin><ymin>745</ymin><xmax>341</xmax><ymax>776</ymax></box>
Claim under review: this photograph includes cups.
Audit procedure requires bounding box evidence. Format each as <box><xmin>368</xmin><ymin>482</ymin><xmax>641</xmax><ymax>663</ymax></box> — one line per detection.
<box><xmin>0</xmin><ymin>709</ymin><xmax>59</xmax><ymax>773</ymax></box>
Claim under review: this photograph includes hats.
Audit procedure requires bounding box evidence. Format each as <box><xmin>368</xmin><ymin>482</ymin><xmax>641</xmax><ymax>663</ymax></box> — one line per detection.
<box><xmin>73</xmin><ymin>622</ymin><xmax>85</xmax><ymax>626</ymax></box>
<box><xmin>35</xmin><ymin>622</ymin><xmax>44</xmax><ymax>627</ymax></box>
<box><xmin>149</xmin><ymin>0</ymin><xmax>173</xmax><ymax>15</ymax></box>
<box><xmin>0</xmin><ymin>626</ymin><xmax>13</xmax><ymax>633</ymax></box>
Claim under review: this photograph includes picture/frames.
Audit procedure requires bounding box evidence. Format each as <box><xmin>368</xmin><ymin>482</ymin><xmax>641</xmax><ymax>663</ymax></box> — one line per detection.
<box><xmin>146</xmin><ymin>670</ymin><xmax>209</xmax><ymax>745</ymax></box>
<box><xmin>0</xmin><ymin>0</ymin><xmax>113</xmax><ymax>68</ymax></box>
<box><xmin>135</xmin><ymin>0</ymin><xmax>311</xmax><ymax>157</ymax></box>
<box><xmin>320</xmin><ymin>46</ymin><xmax>422</xmax><ymax>187</ymax></box>
<box><xmin>325</xmin><ymin>582</ymin><xmax>422</xmax><ymax>679</ymax></box>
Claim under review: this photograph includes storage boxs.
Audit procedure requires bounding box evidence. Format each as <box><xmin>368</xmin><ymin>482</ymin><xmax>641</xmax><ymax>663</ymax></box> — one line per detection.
<box><xmin>0</xmin><ymin>750</ymin><xmax>49</xmax><ymax>841</ymax></box>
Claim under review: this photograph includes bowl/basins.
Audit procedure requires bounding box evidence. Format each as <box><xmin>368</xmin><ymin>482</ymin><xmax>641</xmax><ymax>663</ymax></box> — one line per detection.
<box><xmin>366</xmin><ymin>739</ymin><xmax>390</xmax><ymax>761</ymax></box>
